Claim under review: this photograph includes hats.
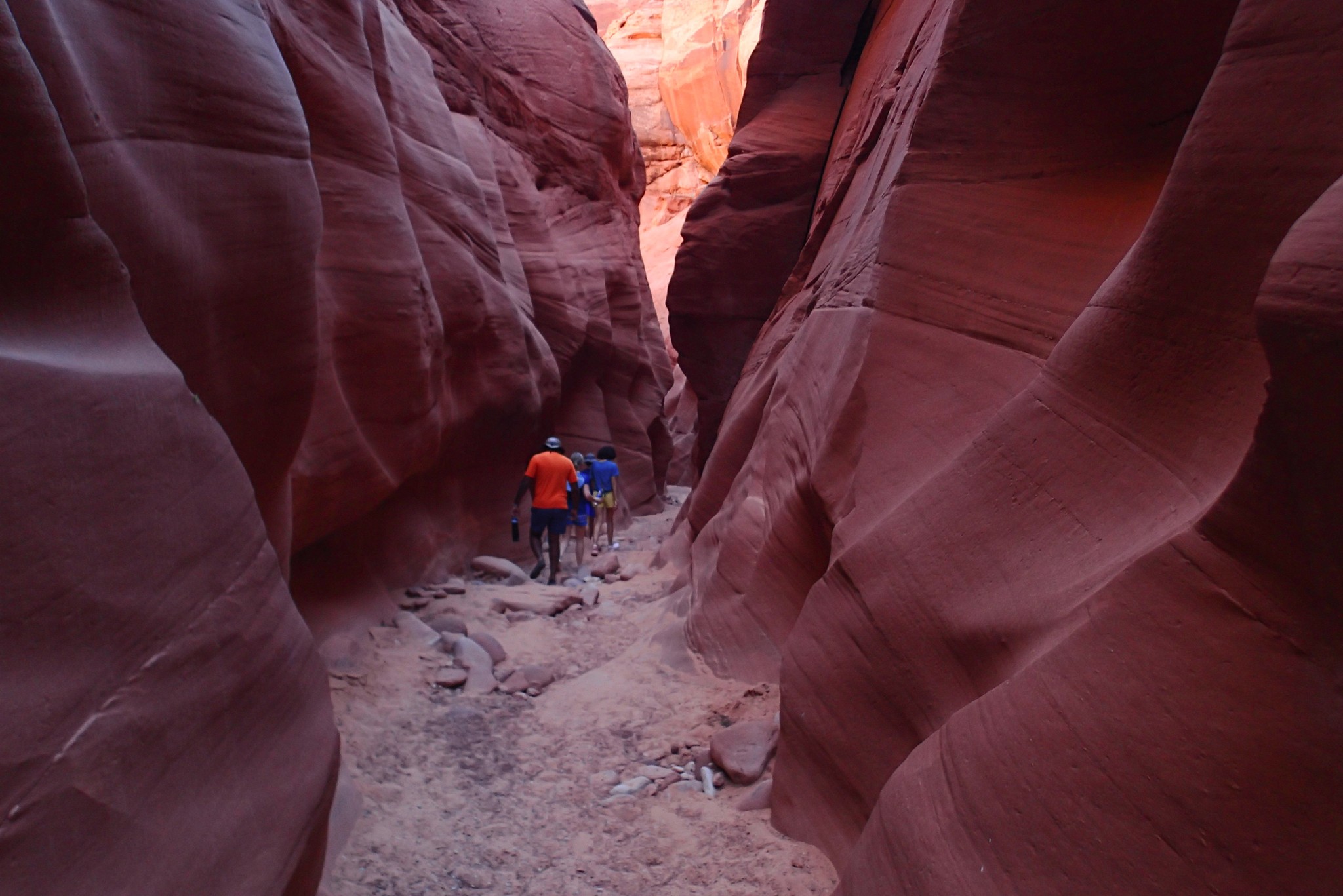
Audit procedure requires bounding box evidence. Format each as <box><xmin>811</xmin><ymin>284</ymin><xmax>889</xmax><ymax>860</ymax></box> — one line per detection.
<box><xmin>546</xmin><ymin>437</ymin><xmax>560</xmax><ymax>449</ymax></box>
<box><xmin>584</xmin><ymin>453</ymin><xmax>597</xmax><ymax>463</ymax></box>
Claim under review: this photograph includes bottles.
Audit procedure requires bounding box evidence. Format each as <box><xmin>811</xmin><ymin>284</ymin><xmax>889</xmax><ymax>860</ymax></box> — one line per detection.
<box><xmin>592</xmin><ymin>489</ymin><xmax>604</xmax><ymax>507</ymax></box>
<box><xmin>512</xmin><ymin>517</ymin><xmax>519</xmax><ymax>543</ymax></box>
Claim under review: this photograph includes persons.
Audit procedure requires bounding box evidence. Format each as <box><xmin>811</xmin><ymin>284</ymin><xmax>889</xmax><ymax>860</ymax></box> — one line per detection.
<box><xmin>511</xmin><ymin>437</ymin><xmax>579</xmax><ymax>586</ymax></box>
<box><xmin>590</xmin><ymin>446</ymin><xmax>620</xmax><ymax>557</ymax></box>
<box><xmin>584</xmin><ymin>453</ymin><xmax>603</xmax><ymax>547</ymax></box>
<box><xmin>559</xmin><ymin>452</ymin><xmax>601</xmax><ymax>572</ymax></box>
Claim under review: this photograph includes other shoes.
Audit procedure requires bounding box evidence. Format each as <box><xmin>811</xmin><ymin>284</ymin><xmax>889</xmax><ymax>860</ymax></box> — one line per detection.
<box><xmin>591</xmin><ymin>545</ymin><xmax>598</xmax><ymax>556</ymax></box>
<box><xmin>530</xmin><ymin>562</ymin><xmax>545</xmax><ymax>579</ymax></box>
<box><xmin>547</xmin><ymin>580</ymin><xmax>556</xmax><ymax>585</ymax></box>
<box><xmin>608</xmin><ymin>543</ymin><xmax>619</xmax><ymax>551</ymax></box>
